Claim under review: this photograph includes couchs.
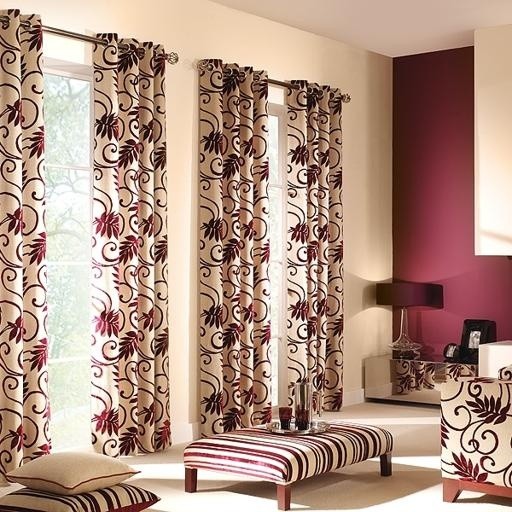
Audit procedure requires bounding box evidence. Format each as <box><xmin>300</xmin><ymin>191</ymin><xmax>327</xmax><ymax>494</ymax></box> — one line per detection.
<box><xmin>437</xmin><ymin>366</ymin><xmax>512</xmax><ymax>503</ymax></box>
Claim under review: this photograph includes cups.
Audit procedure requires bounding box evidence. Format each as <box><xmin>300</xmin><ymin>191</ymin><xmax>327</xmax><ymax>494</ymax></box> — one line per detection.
<box><xmin>295</xmin><ymin>409</ymin><xmax>309</xmax><ymax>430</ymax></box>
<box><xmin>278</xmin><ymin>406</ymin><xmax>292</xmax><ymax>429</ymax></box>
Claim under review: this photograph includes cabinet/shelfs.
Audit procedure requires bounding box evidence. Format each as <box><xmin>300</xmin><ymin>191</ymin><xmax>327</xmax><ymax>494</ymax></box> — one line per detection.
<box><xmin>478</xmin><ymin>340</ymin><xmax>512</xmax><ymax>377</ymax></box>
<box><xmin>365</xmin><ymin>355</ymin><xmax>477</xmax><ymax>405</ymax></box>
<box><xmin>473</xmin><ymin>24</ymin><xmax>512</xmax><ymax>256</ymax></box>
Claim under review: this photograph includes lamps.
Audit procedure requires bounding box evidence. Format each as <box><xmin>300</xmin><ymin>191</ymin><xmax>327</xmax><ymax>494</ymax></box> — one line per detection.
<box><xmin>376</xmin><ymin>282</ymin><xmax>444</xmax><ymax>352</ymax></box>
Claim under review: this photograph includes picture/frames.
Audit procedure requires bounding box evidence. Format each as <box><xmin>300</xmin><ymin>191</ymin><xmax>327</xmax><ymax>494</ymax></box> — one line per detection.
<box><xmin>461</xmin><ymin>319</ymin><xmax>496</xmax><ymax>364</ymax></box>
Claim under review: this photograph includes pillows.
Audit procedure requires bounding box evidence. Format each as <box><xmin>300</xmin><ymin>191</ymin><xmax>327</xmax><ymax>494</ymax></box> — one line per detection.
<box><xmin>5</xmin><ymin>452</ymin><xmax>142</xmax><ymax>495</ymax></box>
<box><xmin>0</xmin><ymin>483</ymin><xmax>161</xmax><ymax>512</ymax></box>
